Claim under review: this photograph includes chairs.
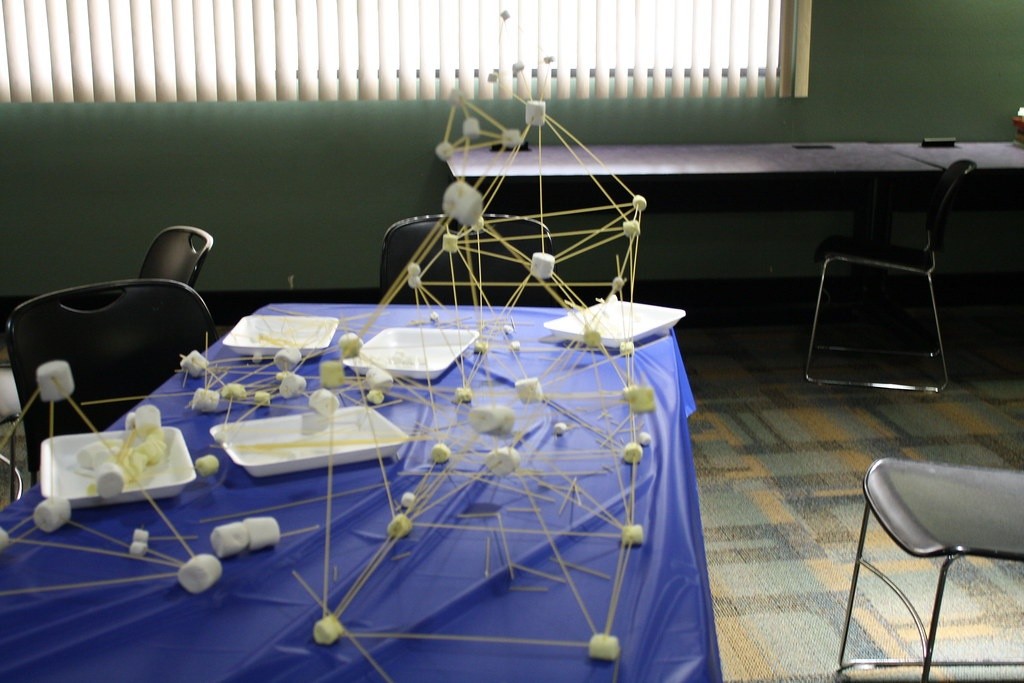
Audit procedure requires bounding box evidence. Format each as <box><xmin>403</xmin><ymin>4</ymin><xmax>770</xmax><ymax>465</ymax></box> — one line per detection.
<box><xmin>835</xmin><ymin>457</ymin><xmax>1024</xmax><ymax>683</ymax></box>
<box><xmin>804</xmin><ymin>159</ymin><xmax>976</xmax><ymax>394</ymax></box>
<box><xmin>377</xmin><ymin>213</ymin><xmax>553</xmax><ymax>307</ymax></box>
<box><xmin>136</xmin><ymin>225</ymin><xmax>214</xmax><ymax>289</ymax></box>
<box><xmin>8</xmin><ymin>279</ymin><xmax>217</xmax><ymax>489</ymax></box>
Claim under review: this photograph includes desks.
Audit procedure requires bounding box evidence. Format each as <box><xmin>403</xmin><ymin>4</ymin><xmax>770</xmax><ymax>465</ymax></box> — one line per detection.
<box><xmin>435</xmin><ymin>142</ymin><xmax>947</xmax><ymax>358</ymax></box>
<box><xmin>874</xmin><ymin>142</ymin><xmax>1024</xmax><ymax>341</ymax></box>
<box><xmin>0</xmin><ymin>304</ymin><xmax>726</xmax><ymax>683</ymax></box>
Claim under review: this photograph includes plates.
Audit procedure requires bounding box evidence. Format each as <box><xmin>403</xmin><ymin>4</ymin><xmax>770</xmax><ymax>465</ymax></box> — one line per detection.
<box><xmin>544</xmin><ymin>301</ymin><xmax>686</xmax><ymax>348</ymax></box>
<box><xmin>343</xmin><ymin>327</ymin><xmax>480</xmax><ymax>379</ymax></box>
<box><xmin>224</xmin><ymin>315</ymin><xmax>340</xmax><ymax>357</ymax></box>
<box><xmin>210</xmin><ymin>405</ymin><xmax>409</xmax><ymax>477</ymax></box>
<box><xmin>41</xmin><ymin>427</ymin><xmax>197</xmax><ymax>509</ymax></box>
